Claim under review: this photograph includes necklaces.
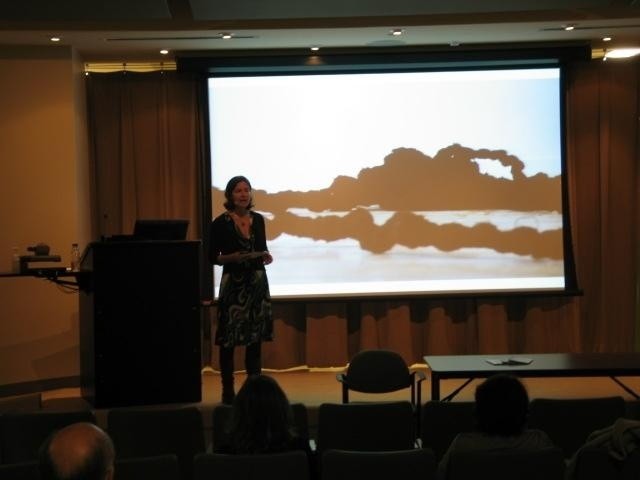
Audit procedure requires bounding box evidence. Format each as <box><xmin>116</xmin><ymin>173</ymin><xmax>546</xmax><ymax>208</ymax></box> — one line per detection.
<box><xmin>237</xmin><ymin>216</ymin><xmax>250</xmax><ymax>226</ymax></box>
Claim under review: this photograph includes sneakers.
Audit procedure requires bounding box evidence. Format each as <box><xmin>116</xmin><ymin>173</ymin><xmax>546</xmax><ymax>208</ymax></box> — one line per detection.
<box><xmin>222</xmin><ymin>382</ymin><xmax>234</xmax><ymax>404</ymax></box>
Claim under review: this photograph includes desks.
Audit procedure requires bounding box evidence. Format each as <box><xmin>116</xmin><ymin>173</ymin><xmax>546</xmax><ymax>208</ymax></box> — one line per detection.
<box><xmin>423</xmin><ymin>353</ymin><xmax>640</xmax><ymax>401</ymax></box>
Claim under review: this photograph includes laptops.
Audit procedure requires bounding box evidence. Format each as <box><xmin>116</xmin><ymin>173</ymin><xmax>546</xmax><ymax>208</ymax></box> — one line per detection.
<box><xmin>134</xmin><ymin>220</ymin><xmax>189</xmax><ymax>240</ymax></box>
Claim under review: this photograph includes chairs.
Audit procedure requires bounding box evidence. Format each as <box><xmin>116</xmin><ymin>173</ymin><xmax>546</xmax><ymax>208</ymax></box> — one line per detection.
<box><xmin>337</xmin><ymin>349</ymin><xmax>426</xmax><ymax>438</ymax></box>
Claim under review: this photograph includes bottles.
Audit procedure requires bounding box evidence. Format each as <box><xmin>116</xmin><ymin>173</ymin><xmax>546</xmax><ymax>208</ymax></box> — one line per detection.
<box><xmin>71</xmin><ymin>244</ymin><xmax>81</xmax><ymax>272</ymax></box>
<box><xmin>11</xmin><ymin>247</ymin><xmax>20</xmax><ymax>274</ymax></box>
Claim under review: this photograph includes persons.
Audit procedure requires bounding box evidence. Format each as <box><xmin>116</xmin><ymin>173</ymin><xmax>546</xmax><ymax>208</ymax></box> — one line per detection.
<box><xmin>37</xmin><ymin>421</ymin><xmax>115</xmax><ymax>480</ymax></box>
<box><xmin>435</xmin><ymin>375</ymin><xmax>553</xmax><ymax>478</ymax></box>
<box><xmin>209</xmin><ymin>176</ymin><xmax>273</xmax><ymax>405</ymax></box>
<box><xmin>207</xmin><ymin>374</ymin><xmax>314</xmax><ymax>463</ymax></box>
